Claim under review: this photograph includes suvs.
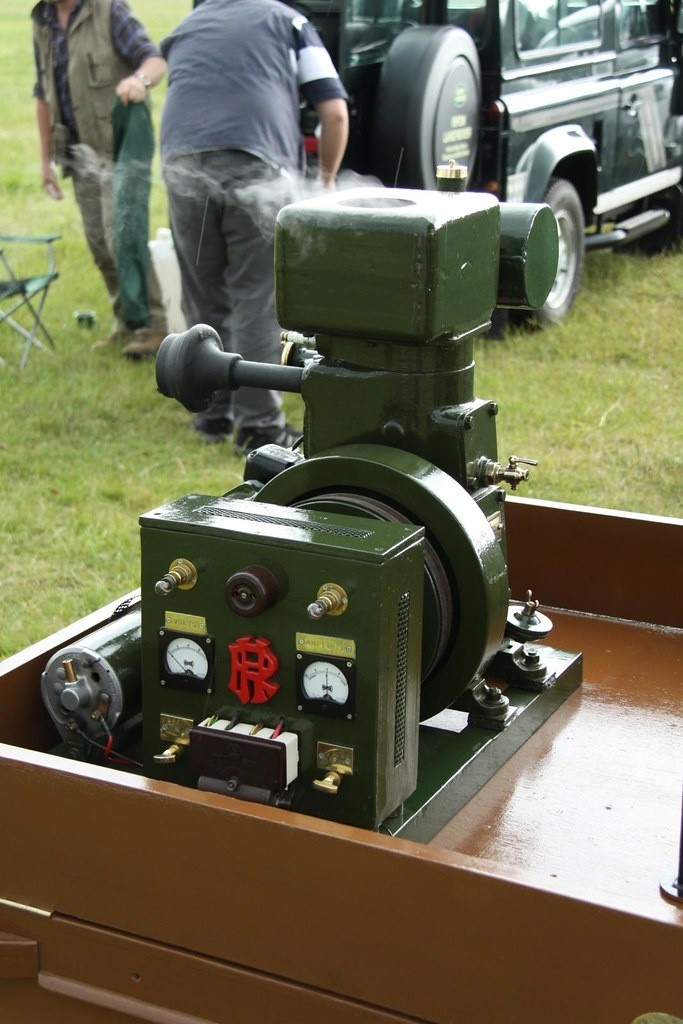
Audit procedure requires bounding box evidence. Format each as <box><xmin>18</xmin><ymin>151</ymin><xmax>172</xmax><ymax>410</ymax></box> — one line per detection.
<box><xmin>192</xmin><ymin>0</ymin><xmax>682</xmax><ymax>340</ymax></box>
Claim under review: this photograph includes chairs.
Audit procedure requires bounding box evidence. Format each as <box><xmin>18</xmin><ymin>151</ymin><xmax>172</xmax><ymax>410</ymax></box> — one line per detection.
<box><xmin>0</xmin><ymin>234</ymin><xmax>61</xmax><ymax>371</ymax></box>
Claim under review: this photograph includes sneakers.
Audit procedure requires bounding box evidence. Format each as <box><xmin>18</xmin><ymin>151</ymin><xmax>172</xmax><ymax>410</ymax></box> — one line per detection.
<box><xmin>92</xmin><ymin>319</ymin><xmax>167</xmax><ymax>356</ymax></box>
<box><xmin>191</xmin><ymin>415</ymin><xmax>233</xmax><ymax>443</ymax></box>
<box><xmin>233</xmin><ymin>423</ymin><xmax>304</xmax><ymax>456</ymax></box>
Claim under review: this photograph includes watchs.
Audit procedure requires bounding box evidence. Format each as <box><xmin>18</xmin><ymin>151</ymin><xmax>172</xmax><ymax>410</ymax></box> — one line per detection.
<box><xmin>133</xmin><ymin>71</ymin><xmax>151</xmax><ymax>90</ymax></box>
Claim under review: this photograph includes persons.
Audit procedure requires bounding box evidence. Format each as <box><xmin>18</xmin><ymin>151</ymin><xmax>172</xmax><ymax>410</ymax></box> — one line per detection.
<box><xmin>160</xmin><ymin>0</ymin><xmax>349</xmax><ymax>459</ymax></box>
<box><xmin>31</xmin><ymin>1</ymin><xmax>167</xmax><ymax>360</ymax></box>
